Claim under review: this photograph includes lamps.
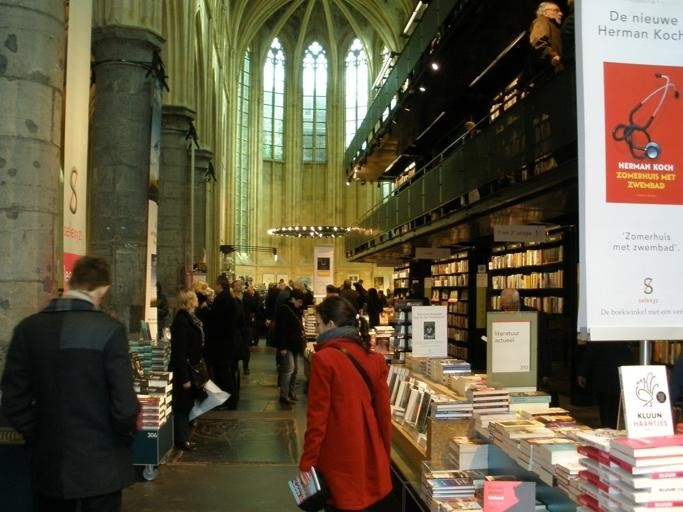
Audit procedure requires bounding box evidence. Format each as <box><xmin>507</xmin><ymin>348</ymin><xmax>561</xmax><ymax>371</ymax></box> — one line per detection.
<box><xmin>346</xmin><ymin>58</ymin><xmax>444</xmax><ymax>188</ymax></box>
<box><xmin>266</xmin><ymin>226</ymin><xmax>352</xmax><ymax>239</ymax></box>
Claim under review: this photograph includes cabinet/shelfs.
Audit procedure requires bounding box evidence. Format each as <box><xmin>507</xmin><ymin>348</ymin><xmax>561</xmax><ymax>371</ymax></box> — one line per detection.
<box><xmin>429</xmin><ymin>251</ymin><xmax>486</xmax><ymax>375</ymax></box>
<box><xmin>392</xmin><ymin>258</ymin><xmax>435</xmax><ymax>307</ymax></box>
<box><xmin>487</xmin><ymin>225</ymin><xmax>627</xmax><ymax>430</ymax></box>
<box><xmin>130</xmin><ymin>416</ymin><xmax>173</xmax><ymax>481</ymax></box>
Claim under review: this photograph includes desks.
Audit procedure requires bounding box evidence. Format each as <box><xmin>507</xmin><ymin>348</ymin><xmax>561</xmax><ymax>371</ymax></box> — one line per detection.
<box><xmin>304</xmin><ymin>341</ymin><xmax>593</xmax><ymax>512</ymax></box>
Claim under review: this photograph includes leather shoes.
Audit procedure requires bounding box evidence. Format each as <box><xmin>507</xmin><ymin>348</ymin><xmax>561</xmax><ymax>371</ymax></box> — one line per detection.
<box><xmin>180</xmin><ymin>441</ymin><xmax>197</xmax><ymax>451</ymax></box>
<box><xmin>217</xmin><ymin>405</ymin><xmax>229</xmax><ymax>410</ymax></box>
<box><xmin>280</xmin><ymin>397</ymin><xmax>299</xmax><ymax>405</ymax></box>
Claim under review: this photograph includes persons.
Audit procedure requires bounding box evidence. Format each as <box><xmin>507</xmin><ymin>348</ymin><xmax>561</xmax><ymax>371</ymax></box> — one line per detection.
<box><xmin>157</xmin><ymin>276</ymin><xmax>392</xmax><ymax>451</ymax></box>
<box><xmin>1</xmin><ymin>256</ymin><xmax>140</xmax><ymax>512</ymax></box>
<box><xmin>299</xmin><ymin>296</ymin><xmax>394</xmax><ymax>512</ymax></box>
<box><xmin>528</xmin><ymin>1</ymin><xmax>562</xmax><ymax>66</ymax></box>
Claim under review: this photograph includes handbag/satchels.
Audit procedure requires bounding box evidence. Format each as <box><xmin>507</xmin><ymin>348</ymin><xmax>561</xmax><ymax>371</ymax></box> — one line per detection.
<box><xmin>190</xmin><ymin>362</ymin><xmax>209</xmax><ymax>388</ymax></box>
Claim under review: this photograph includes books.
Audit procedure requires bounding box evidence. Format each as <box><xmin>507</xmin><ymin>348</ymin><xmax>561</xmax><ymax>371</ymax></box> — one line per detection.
<box><xmin>288</xmin><ymin>466</ymin><xmax>321</xmax><ymax>506</ymax></box>
<box><xmin>368</xmin><ymin>244</ymin><xmax>683</xmax><ymax>512</ymax></box>
<box><xmin>128</xmin><ymin>339</ymin><xmax>173</xmax><ymax>431</ymax></box>
